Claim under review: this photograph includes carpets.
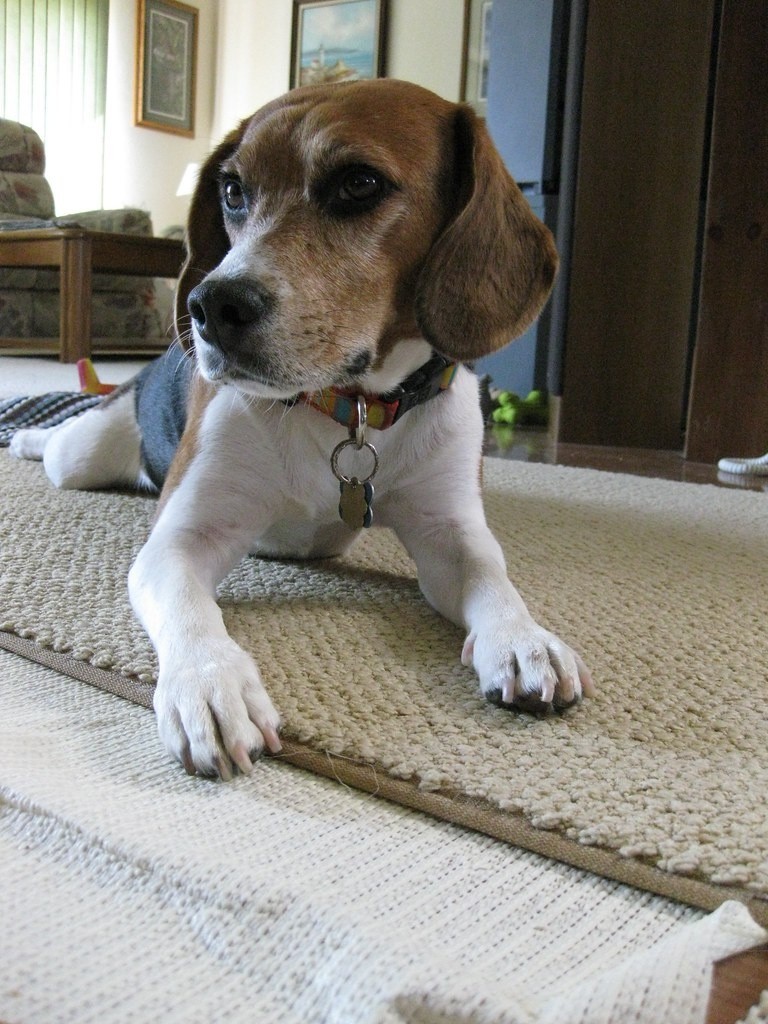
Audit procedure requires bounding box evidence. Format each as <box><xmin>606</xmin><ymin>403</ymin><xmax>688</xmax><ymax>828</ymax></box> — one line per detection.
<box><xmin>0</xmin><ymin>447</ymin><xmax>768</xmax><ymax>931</ymax></box>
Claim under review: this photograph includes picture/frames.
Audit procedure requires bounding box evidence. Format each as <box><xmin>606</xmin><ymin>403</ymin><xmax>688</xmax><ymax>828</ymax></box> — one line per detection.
<box><xmin>133</xmin><ymin>0</ymin><xmax>199</xmax><ymax>139</ymax></box>
<box><xmin>289</xmin><ymin>0</ymin><xmax>390</xmax><ymax>91</ymax></box>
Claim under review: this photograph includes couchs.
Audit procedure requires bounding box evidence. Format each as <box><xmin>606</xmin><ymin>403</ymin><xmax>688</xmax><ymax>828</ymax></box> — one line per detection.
<box><xmin>0</xmin><ymin>116</ymin><xmax>163</xmax><ymax>339</ymax></box>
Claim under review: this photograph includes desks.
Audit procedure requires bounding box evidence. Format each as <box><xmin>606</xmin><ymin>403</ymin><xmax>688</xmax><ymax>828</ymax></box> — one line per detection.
<box><xmin>0</xmin><ymin>228</ymin><xmax>188</xmax><ymax>364</ymax></box>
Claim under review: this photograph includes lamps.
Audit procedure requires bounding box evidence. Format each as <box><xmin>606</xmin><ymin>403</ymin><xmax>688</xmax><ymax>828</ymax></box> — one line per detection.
<box><xmin>176</xmin><ymin>163</ymin><xmax>202</xmax><ymax>196</ymax></box>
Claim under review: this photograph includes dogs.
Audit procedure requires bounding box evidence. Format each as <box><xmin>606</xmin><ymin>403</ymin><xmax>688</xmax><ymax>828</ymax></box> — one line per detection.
<box><xmin>7</xmin><ymin>76</ymin><xmax>595</xmax><ymax>784</ymax></box>
